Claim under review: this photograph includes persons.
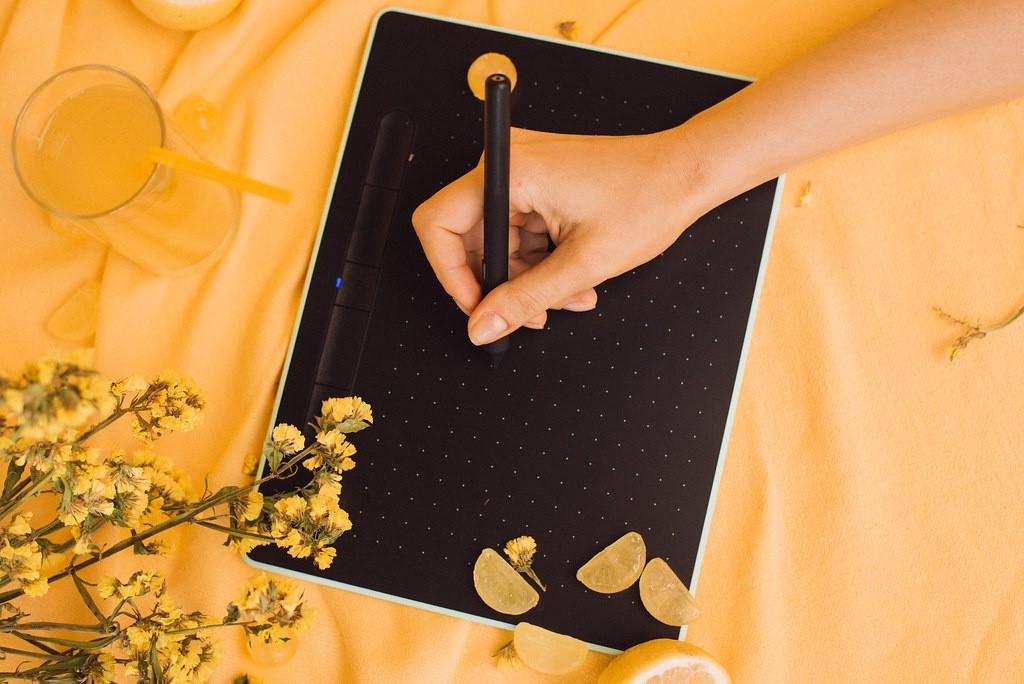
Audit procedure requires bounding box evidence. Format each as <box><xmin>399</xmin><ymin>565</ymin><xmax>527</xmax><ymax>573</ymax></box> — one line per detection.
<box><xmin>411</xmin><ymin>0</ymin><xmax>1024</xmax><ymax>347</ymax></box>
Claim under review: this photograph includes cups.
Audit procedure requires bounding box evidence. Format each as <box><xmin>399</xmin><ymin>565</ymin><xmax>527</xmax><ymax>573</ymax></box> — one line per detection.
<box><xmin>11</xmin><ymin>63</ymin><xmax>240</xmax><ymax>276</ymax></box>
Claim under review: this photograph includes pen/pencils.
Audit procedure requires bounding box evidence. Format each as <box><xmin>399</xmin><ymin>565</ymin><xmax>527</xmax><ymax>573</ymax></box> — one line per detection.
<box><xmin>481</xmin><ymin>73</ymin><xmax>516</xmax><ymax>373</ymax></box>
